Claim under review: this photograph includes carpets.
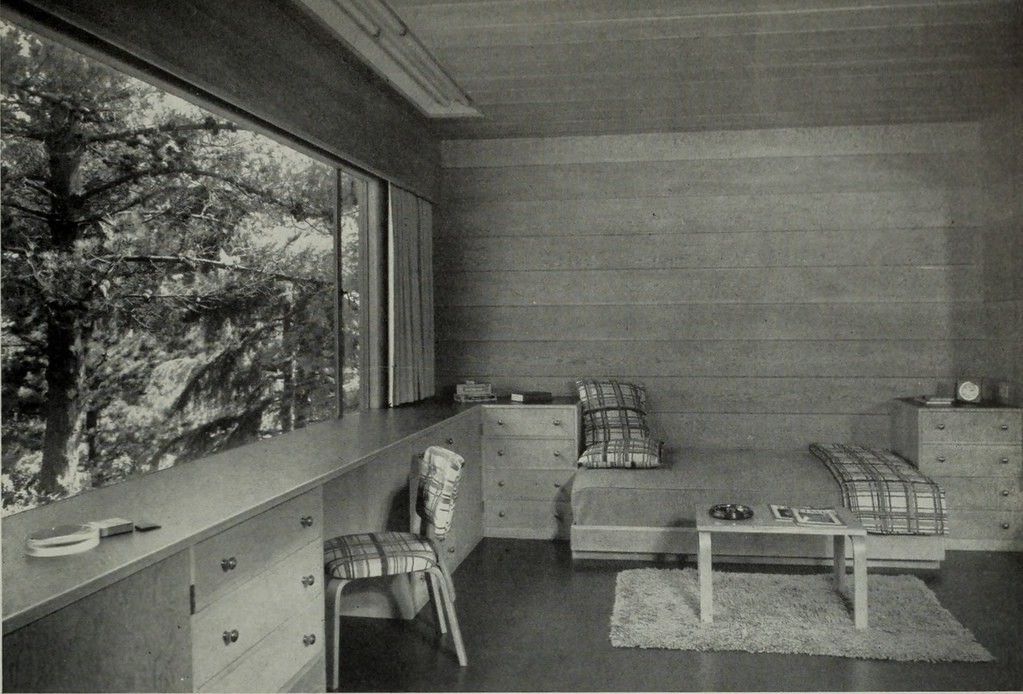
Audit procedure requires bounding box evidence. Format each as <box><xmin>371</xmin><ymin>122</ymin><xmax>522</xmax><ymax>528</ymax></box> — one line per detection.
<box><xmin>609</xmin><ymin>564</ymin><xmax>999</xmax><ymax>663</ymax></box>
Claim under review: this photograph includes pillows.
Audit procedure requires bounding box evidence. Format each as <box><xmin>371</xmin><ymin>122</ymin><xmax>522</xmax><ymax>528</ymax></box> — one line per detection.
<box><xmin>577</xmin><ymin>376</ymin><xmax>664</xmax><ymax>470</ymax></box>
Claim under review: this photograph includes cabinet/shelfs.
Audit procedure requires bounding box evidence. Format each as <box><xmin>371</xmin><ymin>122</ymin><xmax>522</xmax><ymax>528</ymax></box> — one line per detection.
<box><xmin>890</xmin><ymin>399</ymin><xmax>1023</xmax><ymax>551</ymax></box>
<box><xmin>480</xmin><ymin>388</ymin><xmax>582</xmax><ymax>544</ymax></box>
<box><xmin>409</xmin><ymin>408</ymin><xmax>481</xmax><ymax>615</ymax></box>
<box><xmin>188</xmin><ymin>487</ymin><xmax>319</xmax><ymax>694</ymax></box>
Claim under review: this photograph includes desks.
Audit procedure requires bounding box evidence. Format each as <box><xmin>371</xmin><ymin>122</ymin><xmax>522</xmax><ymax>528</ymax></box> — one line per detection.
<box><xmin>695</xmin><ymin>500</ymin><xmax>871</xmax><ymax>630</ymax></box>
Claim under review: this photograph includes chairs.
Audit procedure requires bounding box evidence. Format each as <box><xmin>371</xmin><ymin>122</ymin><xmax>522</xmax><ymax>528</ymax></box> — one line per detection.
<box><xmin>321</xmin><ymin>444</ymin><xmax>471</xmax><ymax>690</ymax></box>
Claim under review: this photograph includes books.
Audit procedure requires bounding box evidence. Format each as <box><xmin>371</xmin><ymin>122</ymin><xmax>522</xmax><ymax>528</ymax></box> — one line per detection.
<box><xmin>510</xmin><ymin>390</ymin><xmax>551</xmax><ymax>405</ymax></box>
<box><xmin>788</xmin><ymin>504</ymin><xmax>847</xmax><ymax>528</ymax></box>
<box><xmin>768</xmin><ymin>504</ymin><xmax>813</xmax><ymax>523</ymax></box>
<box><xmin>924</xmin><ymin>394</ymin><xmax>955</xmax><ymax>402</ymax></box>
<box><xmin>914</xmin><ymin>397</ymin><xmax>951</xmax><ymax>406</ymax></box>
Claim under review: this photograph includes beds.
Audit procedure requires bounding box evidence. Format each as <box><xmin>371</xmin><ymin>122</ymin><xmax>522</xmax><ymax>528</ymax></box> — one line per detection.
<box><xmin>568</xmin><ymin>445</ymin><xmax>948</xmax><ymax>571</ymax></box>
